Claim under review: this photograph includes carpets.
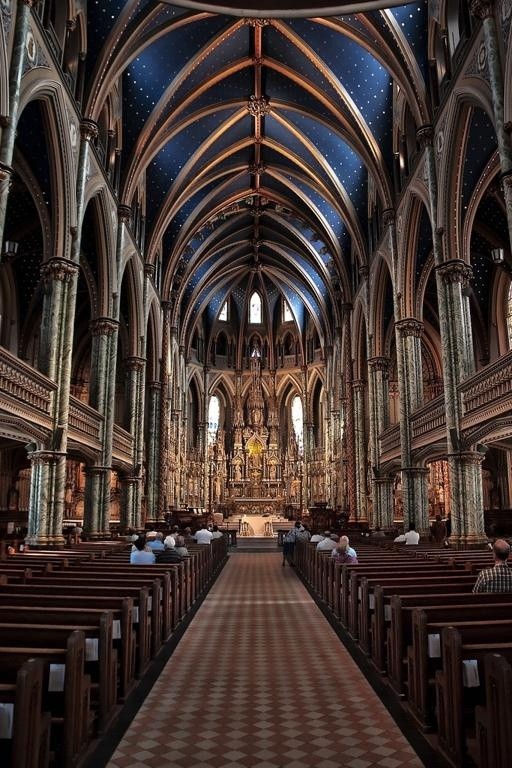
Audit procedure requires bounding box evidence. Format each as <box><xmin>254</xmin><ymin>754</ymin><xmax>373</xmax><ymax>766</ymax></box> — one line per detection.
<box><xmin>104</xmin><ymin>552</ymin><xmax>425</xmax><ymax>768</ymax></box>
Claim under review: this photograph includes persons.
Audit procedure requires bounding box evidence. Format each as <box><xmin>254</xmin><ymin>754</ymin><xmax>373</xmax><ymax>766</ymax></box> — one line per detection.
<box><xmin>393</xmin><ymin>522</ymin><xmax>421</xmax><ymax>545</ymax></box>
<box><xmin>310</xmin><ymin>530</ymin><xmax>325</xmax><ymax>542</ymax></box>
<box><xmin>123</xmin><ymin>523</ymin><xmax>224</xmax><ymax>564</ymax></box>
<box><xmin>471</xmin><ymin>539</ymin><xmax>512</xmax><ymax>594</ymax></box>
<box><xmin>331</xmin><ymin>534</ymin><xmax>359</xmax><ymax>564</ymax></box>
<box><xmin>285</xmin><ymin>521</ymin><xmax>301</xmax><ymax>542</ymax></box>
<box><xmin>431</xmin><ymin>514</ymin><xmax>448</xmax><ymax>542</ymax></box>
<box><xmin>316</xmin><ymin>529</ymin><xmax>339</xmax><ymax>550</ymax></box>
<box><xmin>446</xmin><ymin>512</ymin><xmax>451</xmax><ymax>537</ymax></box>
<box><xmin>296</xmin><ymin>524</ymin><xmax>310</xmax><ymax>542</ymax></box>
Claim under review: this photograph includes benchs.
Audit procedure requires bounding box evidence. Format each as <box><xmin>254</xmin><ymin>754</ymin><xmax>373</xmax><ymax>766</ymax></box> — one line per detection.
<box><xmin>294</xmin><ymin>534</ymin><xmax>510</xmax><ymax>768</ymax></box>
<box><xmin>1</xmin><ymin>530</ymin><xmax>227</xmax><ymax>768</ymax></box>
